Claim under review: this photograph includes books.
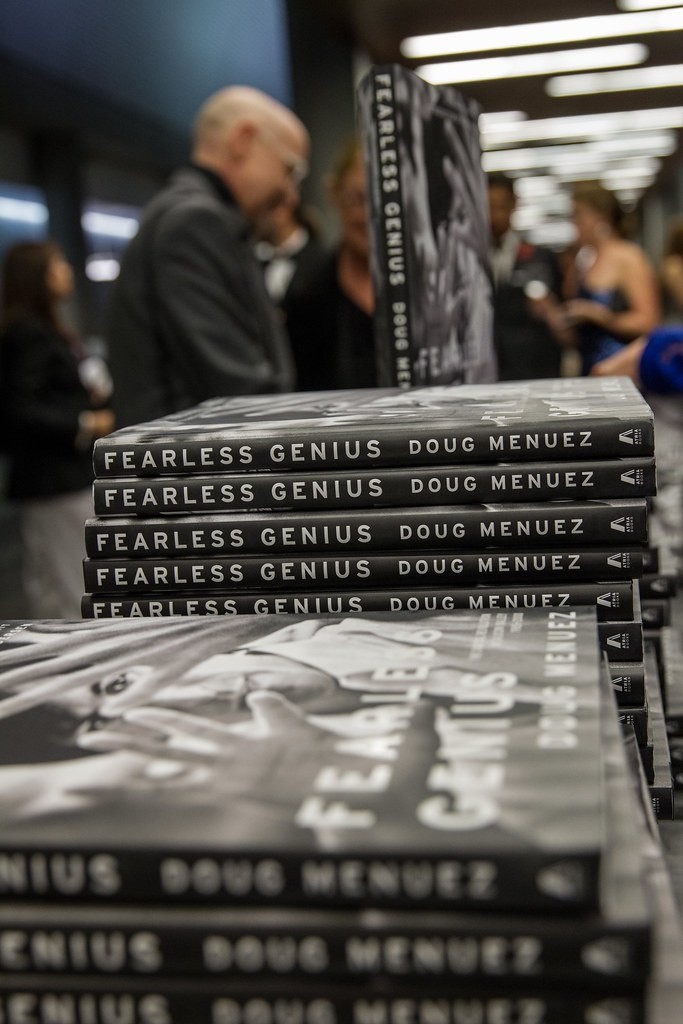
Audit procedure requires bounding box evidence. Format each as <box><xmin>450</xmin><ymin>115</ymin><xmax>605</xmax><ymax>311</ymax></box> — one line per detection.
<box><xmin>0</xmin><ymin>377</ymin><xmax>683</xmax><ymax>1024</ymax></box>
<box><xmin>357</xmin><ymin>62</ymin><xmax>497</xmax><ymax>389</ymax></box>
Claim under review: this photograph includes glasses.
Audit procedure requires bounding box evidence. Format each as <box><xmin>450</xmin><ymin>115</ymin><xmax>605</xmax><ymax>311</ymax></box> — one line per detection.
<box><xmin>253</xmin><ymin>121</ymin><xmax>307</xmax><ymax>183</ymax></box>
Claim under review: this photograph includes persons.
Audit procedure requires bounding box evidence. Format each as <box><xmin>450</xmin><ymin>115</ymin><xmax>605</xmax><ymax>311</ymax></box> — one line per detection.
<box><xmin>486</xmin><ymin>170</ymin><xmax>683</xmax><ymax>395</ymax></box>
<box><xmin>165</xmin><ymin>392</ymin><xmax>479</xmax><ymax>427</ymax></box>
<box><xmin>0</xmin><ymin>244</ymin><xmax>113</xmax><ymax>503</ymax></box>
<box><xmin>112</xmin><ymin>84</ymin><xmax>377</xmax><ymax>429</ymax></box>
<box><xmin>397</xmin><ymin>94</ymin><xmax>493</xmax><ymax>384</ymax></box>
<box><xmin>0</xmin><ymin>618</ymin><xmax>411</xmax><ymax>784</ymax></box>
